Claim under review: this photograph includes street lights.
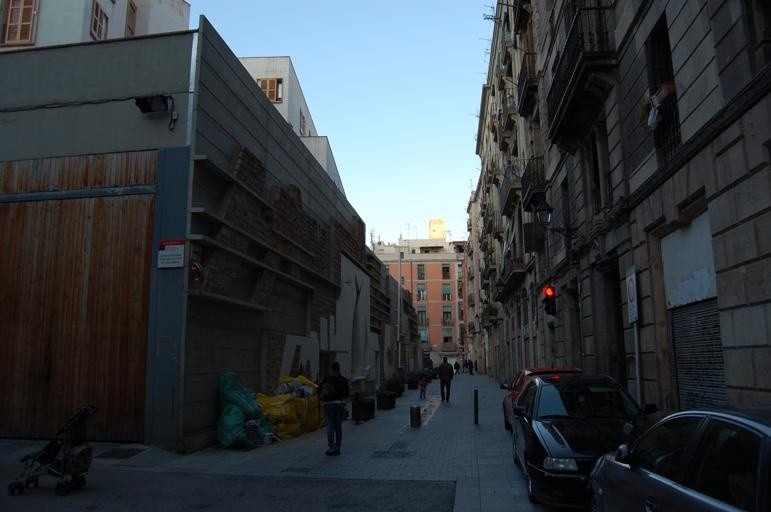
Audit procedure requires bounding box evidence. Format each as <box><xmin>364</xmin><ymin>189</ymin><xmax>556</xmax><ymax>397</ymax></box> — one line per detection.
<box><xmin>396</xmin><ymin>249</ymin><xmax>404</xmax><ymax>342</ymax></box>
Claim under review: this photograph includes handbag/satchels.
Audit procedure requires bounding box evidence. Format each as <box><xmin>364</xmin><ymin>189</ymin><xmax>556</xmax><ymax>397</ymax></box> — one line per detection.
<box><xmin>319</xmin><ymin>383</ymin><xmax>335</xmax><ymax>402</ymax></box>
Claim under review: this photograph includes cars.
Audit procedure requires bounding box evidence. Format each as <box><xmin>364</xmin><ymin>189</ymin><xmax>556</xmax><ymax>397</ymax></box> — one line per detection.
<box><xmin>585</xmin><ymin>406</ymin><xmax>770</xmax><ymax>511</ymax></box>
<box><xmin>500</xmin><ymin>367</ymin><xmax>582</xmax><ymax>430</ymax></box>
<box><xmin>509</xmin><ymin>370</ymin><xmax>657</xmax><ymax>508</ymax></box>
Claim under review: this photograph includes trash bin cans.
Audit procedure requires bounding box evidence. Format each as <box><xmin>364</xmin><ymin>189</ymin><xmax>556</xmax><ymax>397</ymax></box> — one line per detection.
<box><xmin>409</xmin><ymin>405</ymin><xmax>422</xmax><ymax>428</ymax></box>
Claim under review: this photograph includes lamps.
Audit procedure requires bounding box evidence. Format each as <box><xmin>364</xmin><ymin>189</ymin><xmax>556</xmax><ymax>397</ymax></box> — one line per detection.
<box><xmin>535</xmin><ymin>197</ymin><xmax>580</xmax><ymax>240</ymax></box>
<box><xmin>134</xmin><ymin>94</ymin><xmax>178</xmax><ymax>131</ymax></box>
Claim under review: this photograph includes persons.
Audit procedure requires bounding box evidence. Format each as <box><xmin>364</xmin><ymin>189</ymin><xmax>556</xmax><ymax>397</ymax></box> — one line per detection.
<box><xmin>316</xmin><ymin>361</ymin><xmax>350</xmax><ymax>456</ymax></box>
<box><xmin>454</xmin><ymin>359</ymin><xmax>477</xmax><ymax>375</ymax></box>
<box><xmin>437</xmin><ymin>357</ymin><xmax>454</xmax><ymax>401</ymax></box>
<box><xmin>419</xmin><ymin>373</ymin><xmax>428</xmax><ymax>399</ymax></box>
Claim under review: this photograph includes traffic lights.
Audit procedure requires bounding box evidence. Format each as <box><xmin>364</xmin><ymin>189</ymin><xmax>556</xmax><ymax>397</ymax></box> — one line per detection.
<box><xmin>541</xmin><ymin>287</ymin><xmax>554</xmax><ymax>313</ymax></box>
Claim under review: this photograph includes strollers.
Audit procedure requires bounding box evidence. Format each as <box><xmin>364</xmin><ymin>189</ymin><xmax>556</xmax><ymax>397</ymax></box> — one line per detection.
<box><xmin>5</xmin><ymin>402</ymin><xmax>98</xmax><ymax>498</ymax></box>
